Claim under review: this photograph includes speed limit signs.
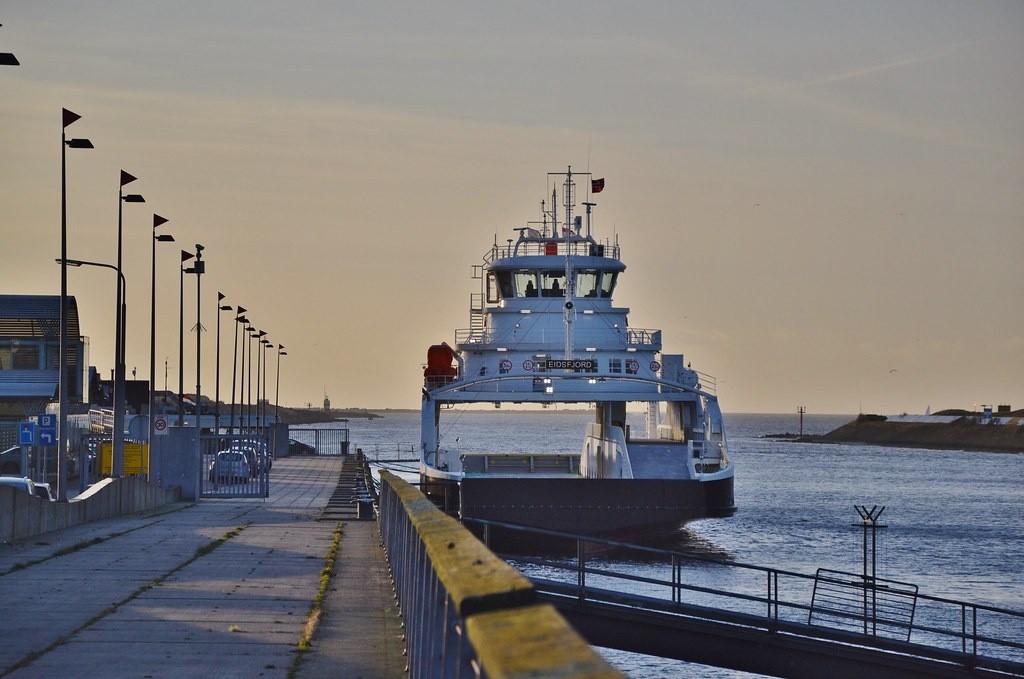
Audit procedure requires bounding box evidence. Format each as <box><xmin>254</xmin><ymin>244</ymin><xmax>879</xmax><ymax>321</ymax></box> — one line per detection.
<box><xmin>154</xmin><ymin>413</ymin><xmax>170</xmax><ymax>436</ymax></box>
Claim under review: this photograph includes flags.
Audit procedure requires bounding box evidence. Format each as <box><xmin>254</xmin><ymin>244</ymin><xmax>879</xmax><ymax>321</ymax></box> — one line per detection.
<box><xmin>592</xmin><ymin>178</ymin><xmax>604</xmax><ymax>193</ymax></box>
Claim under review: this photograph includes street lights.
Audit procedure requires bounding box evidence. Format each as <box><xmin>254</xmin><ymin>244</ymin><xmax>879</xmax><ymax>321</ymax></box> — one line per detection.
<box><xmin>55</xmin><ymin>258</ymin><xmax>128</xmax><ymax>479</ymax></box>
<box><xmin>228</xmin><ymin>438</ymin><xmax>273</xmax><ymax>474</ymax></box>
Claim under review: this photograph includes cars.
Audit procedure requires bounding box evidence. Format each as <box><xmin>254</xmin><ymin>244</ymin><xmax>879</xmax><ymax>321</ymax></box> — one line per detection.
<box><xmin>0</xmin><ymin>445</ymin><xmax>33</xmax><ymax>475</ymax></box>
<box><xmin>208</xmin><ymin>451</ymin><xmax>250</xmax><ymax>485</ymax></box>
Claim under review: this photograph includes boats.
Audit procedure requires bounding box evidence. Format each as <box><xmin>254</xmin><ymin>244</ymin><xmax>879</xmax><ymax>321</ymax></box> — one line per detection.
<box><xmin>418</xmin><ymin>165</ymin><xmax>737</xmax><ymax>543</ymax></box>
